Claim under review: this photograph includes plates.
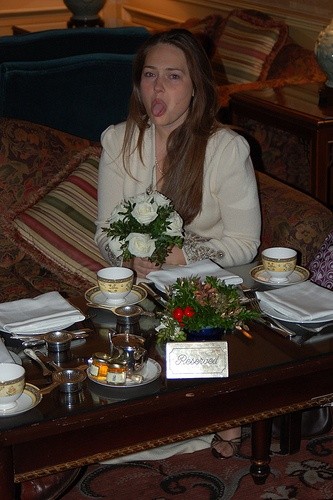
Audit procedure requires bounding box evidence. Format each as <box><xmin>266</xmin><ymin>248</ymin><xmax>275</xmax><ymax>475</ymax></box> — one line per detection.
<box><xmin>0</xmin><ymin>381</ymin><xmax>42</xmax><ymax>417</ymax></box>
<box><xmin>248</xmin><ymin>264</ymin><xmax>310</xmax><ymax>288</ymax></box>
<box><xmin>88</xmin><ymin>358</ymin><xmax>162</xmax><ymax>387</ymax></box>
<box><xmin>84</xmin><ymin>285</ymin><xmax>148</xmax><ymax>307</ymax></box>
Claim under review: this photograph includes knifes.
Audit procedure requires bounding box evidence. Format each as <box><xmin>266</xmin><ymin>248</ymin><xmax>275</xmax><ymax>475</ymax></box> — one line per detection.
<box><xmin>251</xmin><ymin>315</ymin><xmax>291</xmax><ymax>338</ymax></box>
<box><xmin>138</xmin><ymin>281</ymin><xmax>169</xmax><ymax>309</ymax></box>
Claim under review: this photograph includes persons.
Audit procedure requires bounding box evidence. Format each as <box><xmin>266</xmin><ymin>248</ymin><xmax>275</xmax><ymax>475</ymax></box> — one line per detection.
<box><xmin>93</xmin><ymin>28</ymin><xmax>260</xmax><ymax>461</ymax></box>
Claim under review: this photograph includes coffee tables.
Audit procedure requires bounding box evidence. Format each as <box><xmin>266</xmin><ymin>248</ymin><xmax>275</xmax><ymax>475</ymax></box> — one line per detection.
<box><xmin>0</xmin><ymin>261</ymin><xmax>333</xmax><ymax>500</ymax></box>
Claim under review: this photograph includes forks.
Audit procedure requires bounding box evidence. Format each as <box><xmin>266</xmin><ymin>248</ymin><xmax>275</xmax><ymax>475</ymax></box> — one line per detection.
<box><xmin>24</xmin><ymin>349</ymin><xmax>54</xmax><ymax>377</ymax></box>
<box><xmin>249</xmin><ymin>296</ymin><xmax>296</xmax><ymax>336</ymax></box>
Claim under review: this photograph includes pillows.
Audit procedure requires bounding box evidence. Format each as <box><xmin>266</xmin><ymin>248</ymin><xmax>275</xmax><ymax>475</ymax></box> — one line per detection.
<box><xmin>0</xmin><ymin>147</ymin><xmax>114</xmax><ymax>289</ymax></box>
<box><xmin>208</xmin><ymin>8</ymin><xmax>291</xmax><ymax>84</ymax></box>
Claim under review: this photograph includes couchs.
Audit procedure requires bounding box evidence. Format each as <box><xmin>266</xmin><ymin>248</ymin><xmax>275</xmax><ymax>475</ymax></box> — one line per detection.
<box><xmin>0</xmin><ymin>119</ymin><xmax>332</xmax><ymax>353</ymax></box>
<box><xmin>170</xmin><ymin>12</ymin><xmax>323</xmax><ymax>199</ymax></box>
<box><xmin>0</xmin><ymin>26</ymin><xmax>153</xmax><ymax>140</ymax></box>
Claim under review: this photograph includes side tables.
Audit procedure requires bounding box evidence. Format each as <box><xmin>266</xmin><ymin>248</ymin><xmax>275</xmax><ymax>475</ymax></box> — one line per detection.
<box><xmin>12</xmin><ymin>17</ymin><xmax>151</xmax><ymax>35</ymax></box>
<box><xmin>229</xmin><ymin>81</ymin><xmax>332</xmax><ymax>211</ymax></box>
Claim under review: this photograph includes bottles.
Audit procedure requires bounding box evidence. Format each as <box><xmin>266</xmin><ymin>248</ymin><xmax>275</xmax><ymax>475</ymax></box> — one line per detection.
<box><xmin>107</xmin><ymin>304</ymin><xmax>149</xmax><ymax>375</ymax></box>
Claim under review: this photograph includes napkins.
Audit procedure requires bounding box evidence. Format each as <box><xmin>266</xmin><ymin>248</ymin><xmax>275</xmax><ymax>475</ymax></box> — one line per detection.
<box><xmin>148</xmin><ymin>260</ymin><xmax>242</xmax><ymax>291</ymax></box>
<box><xmin>256</xmin><ymin>280</ymin><xmax>332</xmax><ymax>321</ymax></box>
<box><xmin>1</xmin><ymin>291</ymin><xmax>88</xmax><ymax>336</ymax></box>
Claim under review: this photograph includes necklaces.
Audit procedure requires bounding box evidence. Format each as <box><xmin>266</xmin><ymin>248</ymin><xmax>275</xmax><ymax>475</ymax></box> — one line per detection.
<box><xmin>154</xmin><ymin>157</ymin><xmax>167</xmax><ymax>177</ymax></box>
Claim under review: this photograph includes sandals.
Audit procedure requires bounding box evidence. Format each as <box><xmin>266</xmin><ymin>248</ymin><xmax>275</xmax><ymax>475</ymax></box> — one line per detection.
<box><xmin>206</xmin><ymin>426</ymin><xmax>242</xmax><ymax>460</ymax></box>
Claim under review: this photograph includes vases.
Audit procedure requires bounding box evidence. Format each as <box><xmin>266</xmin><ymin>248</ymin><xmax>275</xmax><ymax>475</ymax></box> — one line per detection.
<box><xmin>188</xmin><ymin>324</ymin><xmax>222</xmax><ymax>341</ymax></box>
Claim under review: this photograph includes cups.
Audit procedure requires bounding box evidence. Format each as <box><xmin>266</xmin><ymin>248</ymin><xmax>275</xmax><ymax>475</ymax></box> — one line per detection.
<box><xmin>53</xmin><ymin>369</ymin><xmax>86</xmax><ymax>393</ymax></box>
<box><xmin>0</xmin><ymin>363</ymin><xmax>26</xmax><ymax>411</ymax></box>
<box><xmin>97</xmin><ymin>267</ymin><xmax>134</xmax><ymax>303</ymax></box>
<box><xmin>261</xmin><ymin>247</ymin><xmax>297</xmax><ymax>282</ymax></box>
<box><xmin>43</xmin><ymin>330</ymin><xmax>75</xmax><ymax>352</ymax></box>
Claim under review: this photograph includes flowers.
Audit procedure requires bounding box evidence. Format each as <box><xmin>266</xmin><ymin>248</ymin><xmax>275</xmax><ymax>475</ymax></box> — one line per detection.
<box><xmin>105</xmin><ymin>186</ymin><xmax>185</xmax><ymax>264</ymax></box>
<box><xmin>166</xmin><ymin>274</ymin><xmax>249</xmax><ymax>331</ymax></box>
